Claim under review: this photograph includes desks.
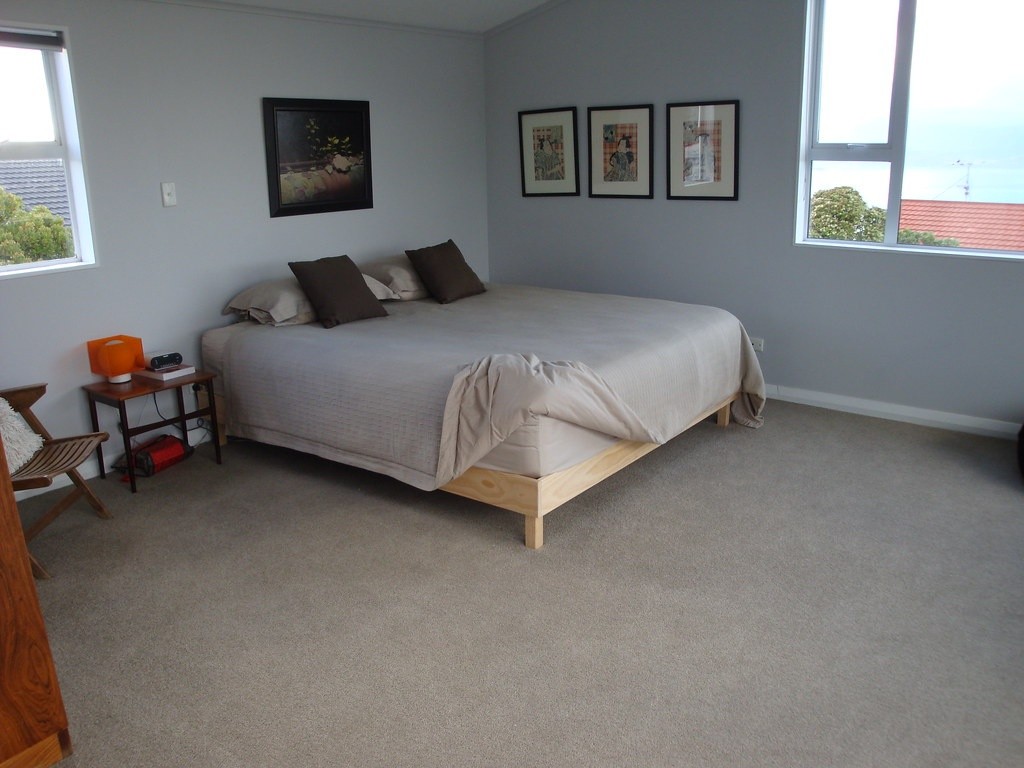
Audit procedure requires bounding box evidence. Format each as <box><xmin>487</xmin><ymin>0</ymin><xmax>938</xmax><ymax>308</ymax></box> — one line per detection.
<box><xmin>80</xmin><ymin>366</ymin><xmax>224</xmax><ymax>493</ymax></box>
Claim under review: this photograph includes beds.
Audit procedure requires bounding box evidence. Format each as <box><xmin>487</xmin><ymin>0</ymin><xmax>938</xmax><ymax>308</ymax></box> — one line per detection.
<box><xmin>200</xmin><ymin>283</ymin><xmax>773</xmax><ymax>552</ymax></box>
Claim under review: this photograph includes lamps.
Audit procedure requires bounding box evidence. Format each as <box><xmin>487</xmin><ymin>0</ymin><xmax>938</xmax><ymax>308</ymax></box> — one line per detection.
<box><xmin>86</xmin><ymin>332</ymin><xmax>147</xmax><ymax>385</ymax></box>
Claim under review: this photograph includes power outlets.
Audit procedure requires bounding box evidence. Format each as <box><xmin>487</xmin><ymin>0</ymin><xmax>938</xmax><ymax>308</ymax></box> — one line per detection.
<box><xmin>749</xmin><ymin>337</ymin><xmax>764</xmax><ymax>352</ymax></box>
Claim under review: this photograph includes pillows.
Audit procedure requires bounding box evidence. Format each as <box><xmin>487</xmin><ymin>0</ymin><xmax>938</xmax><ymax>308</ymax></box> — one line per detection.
<box><xmin>405</xmin><ymin>239</ymin><xmax>487</xmax><ymax>305</ymax></box>
<box><xmin>222</xmin><ymin>275</ymin><xmax>400</xmax><ymax>328</ymax></box>
<box><xmin>369</xmin><ymin>252</ymin><xmax>427</xmax><ymax>300</ymax></box>
<box><xmin>286</xmin><ymin>254</ymin><xmax>389</xmax><ymax>330</ymax></box>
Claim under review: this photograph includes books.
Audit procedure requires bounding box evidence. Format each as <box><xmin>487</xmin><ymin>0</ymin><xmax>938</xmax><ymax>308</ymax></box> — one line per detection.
<box><xmin>133</xmin><ymin>364</ymin><xmax>196</xmax><ymax>381</ymax></box>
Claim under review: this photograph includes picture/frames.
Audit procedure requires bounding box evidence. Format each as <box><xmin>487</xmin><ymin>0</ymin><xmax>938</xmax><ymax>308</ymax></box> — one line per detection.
<box><xmin>517</xmin><ymin>103</ymin><xmax>582</xmax><ymax>199</ymax></box>
<box><xmin>664</xmin><ymin>97</ymin><xmax>741</xmax><ymax>202</ymax></box>
<box><xmin>259</xmin><ymin>94</ymin><xmax>379</xmax><ymax>220</ymax></box>
<box><xmin>587</xmin><ymin>102</ymin><xmax>655</xmax><ymax>200</ymax></box>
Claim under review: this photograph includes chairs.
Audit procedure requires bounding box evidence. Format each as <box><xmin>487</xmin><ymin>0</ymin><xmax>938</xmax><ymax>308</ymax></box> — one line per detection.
<box><xmin>0</xmin><ymin>381</ymin><xmax>114</xmax><ymax>577</ymax></box>
